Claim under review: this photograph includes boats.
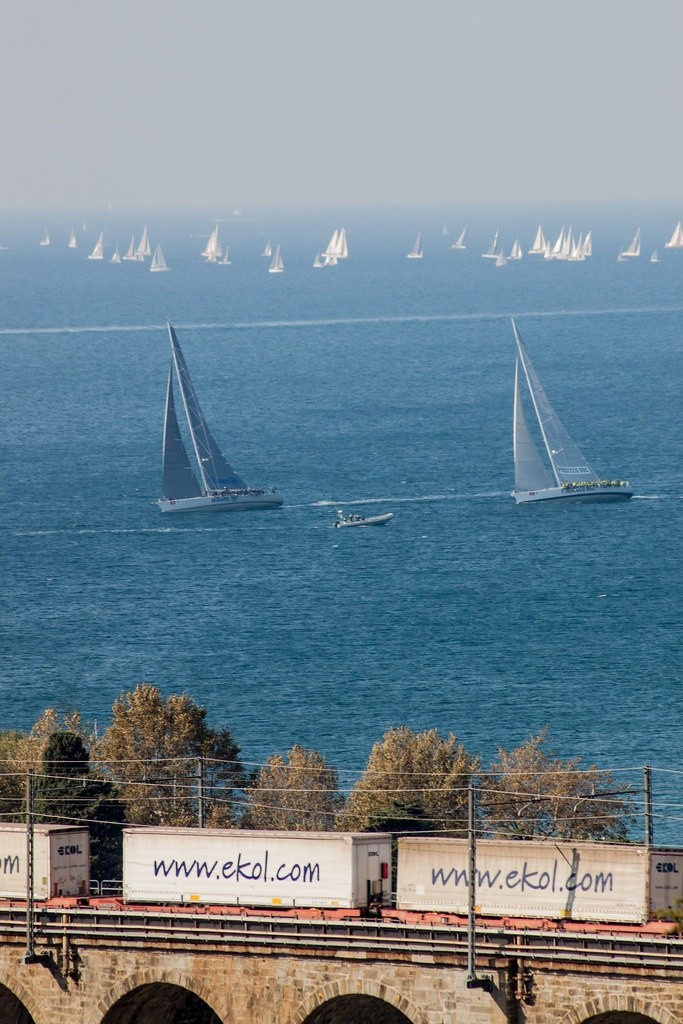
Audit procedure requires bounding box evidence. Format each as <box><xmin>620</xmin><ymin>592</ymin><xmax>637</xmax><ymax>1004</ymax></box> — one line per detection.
<box><xmin>332</xmin><ymin>513</ymin><xmax>394</xmax><ymax>529</ymax></box>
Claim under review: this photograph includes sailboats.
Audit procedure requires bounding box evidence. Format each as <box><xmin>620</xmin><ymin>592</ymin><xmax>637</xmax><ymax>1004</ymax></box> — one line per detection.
<box><xmin>40</xmin><ymin>225</ymin><xmax>171</xmax><ymax>273</ymax></box>
<box><xmin>155</xmin><ymin>317</ymin><xmax>283</xmax><ymax>513</ymax></box>
<box><xmin>510</xmin><ymin>316</ymin><xmax>634</xmax><ymax>504</ymax></box>
<box><xmin>406</xmin><ymin>218</ymin><xmax>682</xmax><ymax>267</ymax></box>
<box><xmin>202</xmin><ymin>224</ymin><xmax>351</xmax><ymax>274</ymax></box>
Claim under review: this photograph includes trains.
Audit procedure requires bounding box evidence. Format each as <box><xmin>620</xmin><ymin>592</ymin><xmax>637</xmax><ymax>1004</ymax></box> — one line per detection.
<box><xmin>0</xmin><ymin>823</ymin><xmax>683</xmax><ymax>927</ymax></box>
